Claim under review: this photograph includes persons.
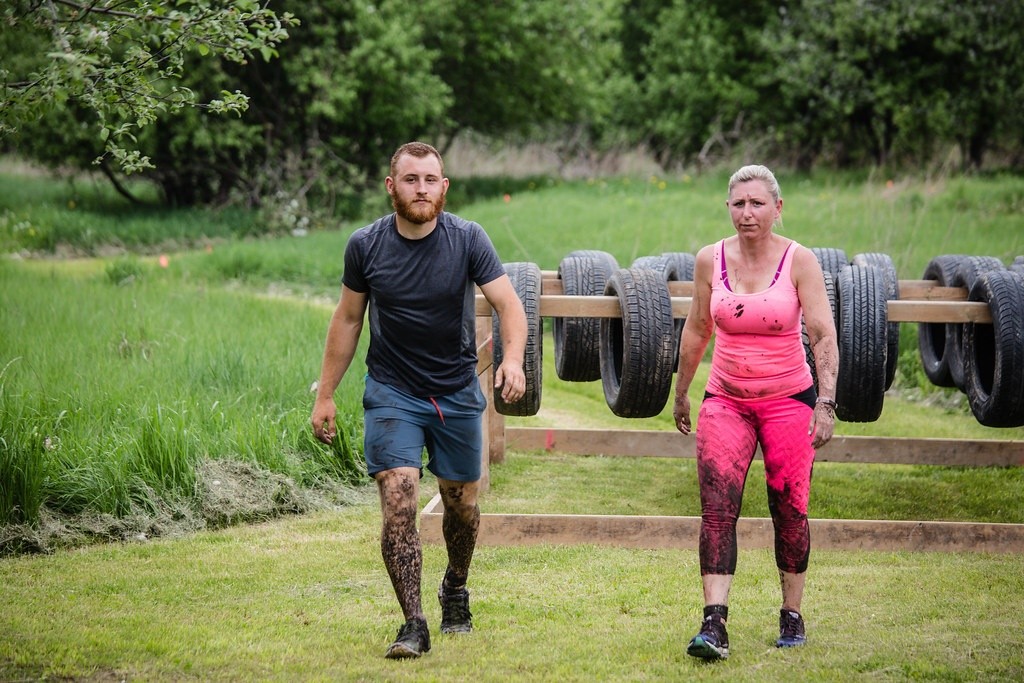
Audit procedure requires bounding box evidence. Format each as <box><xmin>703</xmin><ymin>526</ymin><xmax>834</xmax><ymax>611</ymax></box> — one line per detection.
<box><xmin>673</xmin><ymin>166</ymin><xmax>839</xmax><ymax>660</ymax></box>
<box><xmin>309</xmin><ymin>141</ymin><xmax>528</xmax><ymax>661</ymax></box>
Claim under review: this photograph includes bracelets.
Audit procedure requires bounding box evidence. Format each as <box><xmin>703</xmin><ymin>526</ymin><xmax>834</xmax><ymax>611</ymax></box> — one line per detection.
<box><xmin>815</xmin><ymin>398</ymin><xmax>838</xmax><ymax>410</ymax></box>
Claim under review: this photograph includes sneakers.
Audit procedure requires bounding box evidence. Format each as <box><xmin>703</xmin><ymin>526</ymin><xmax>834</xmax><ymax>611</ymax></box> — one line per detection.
<box><xmin>385</xmin><ymin>618</ymin><xmax>431</xmax><ymax>659</ymax></box>
<box><xmin>775</xmin><ymin>609</ymin><xmax>807</xmax><ymax>646</ymax></box>
<box><xmin>438</xmin><ymin>577</ymin><xmax>473</xmax><ymax>634</ymax></box>
<box><xmin>686</xmin><ymin>614</ymin><xmax>730</xmax><ymax>661</ymax></box>
